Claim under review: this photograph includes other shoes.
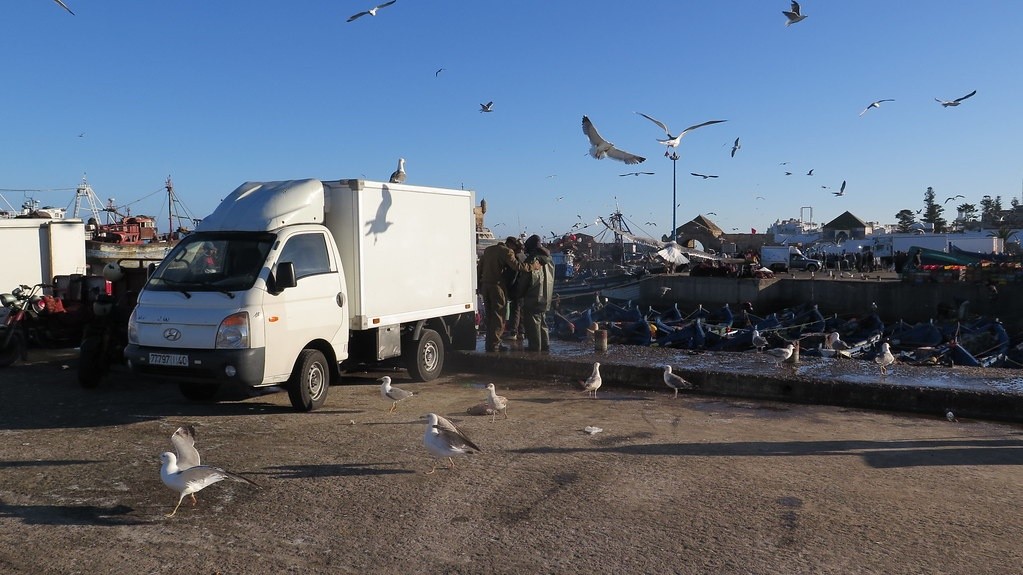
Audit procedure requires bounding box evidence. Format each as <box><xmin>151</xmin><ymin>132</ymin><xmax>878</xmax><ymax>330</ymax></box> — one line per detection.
<box><xmin>517</xmin><ymin>333</ymin><xmax>525</xmax><ymax>339</ymax></box>
<box><xmin>497</xmin><ymin>346</ymin><xmax>509</xmax><ymax>352</ymax></box>
<box><xmin>503</xmin><ymin>334</ymin><xmax>517</xmax><ymax>341</ymax></box>
<box><xmin>524</xmin><ymin>347</ymin><xmax>533</xmax><ymax>352</ymax></box>
<box><xmin>540</xmin><ymin>345</ymin><xmax>550</xmax><ymax>352</ymax></box>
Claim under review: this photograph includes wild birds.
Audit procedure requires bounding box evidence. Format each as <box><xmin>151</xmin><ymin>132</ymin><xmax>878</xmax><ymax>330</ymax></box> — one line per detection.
<box><xmin>831</xmin><ymin>332</ymin><xmax>851</xmax><ymax>359</ymax></box>
<box><xmin>346</xmin><ymin>0</ymin><xmax>398</xmax><ymax>23</ymax></box>
<box><xmin>419</xmin><ymin>413</ymin><xmax>485</xmax><ymax>476</ymax></box>
<box><xmin>662</xmin><ymin>365</ymin><xmax>695</xmax><ymax>401</ymax></box>
<box><xmin>389</xmin><ymin>158</ymin><xmax>407</xmax><ymax>184</ymax></box>
<box><xmin>782</xmin><ymin>0</ymin><xmax>808</xmax><ymax>30</ymax></box>
<box><xmin>77</xmin><ymin>133</ymin><xmax>86</xmax><ymax>137</ymax></box>
<box><xmin>376</xmin><ymin>375</ymin><xmax>421</xmax><ymax>414</ymax></box>
<box><xmin>859</xmin><ymin>98</ymin><xmax>895</xmax><ymax>120</ymax></box>
<box><xmin>581</xmin><ymin>114</ymin><xmax>647</xmax><ymax>165</ymax></box>
<box><xmin>492</xmin><ymin>112</ymin><xmax>1009</xmax><ymax>295</ymax></box>
<box><xmin>933</xmin><ymin>90</ymin><xmax>978</xmax><ymax>108</ymax></box>
<box><xmin>1013</xmin><ymin>234</ymin><xmax>1022</xmax><ymax>245</ymax></box>
<box><xmin>436</xmin><ymin>68</ymin><xmax>447</xmax><ymax>76</ymax></box>
<box><xmin>485</xmin><ymin>382</ymin><xmax>511</xmax><ymax>422</ymax></box>
<box><xmin>874</xmin><ymin>343</ymin><xmax>895</xmax><ymax>374</ymax></box>
<box><xmin>768</xmin><ymin>344</ymin><xmax>797</xmax><ymax>368</ymax></box>
<box><xmin>752</xmin><ymin>331</ymin><xmax>769</xmax><ymax>353</ymax></box>
<box><xmin>579</xmin><ymin>362</ymin><xmax>604</xmax><ymax>400</ymax></box>
<box><xmin>478</xmin><ymin>101</ymin><xmax>495</xmax><ymax>113</ymax></box>
<box><xmin>158</xmin><ymin>425</ymin><xmax>265</xmax><ymax>517</ymax></box>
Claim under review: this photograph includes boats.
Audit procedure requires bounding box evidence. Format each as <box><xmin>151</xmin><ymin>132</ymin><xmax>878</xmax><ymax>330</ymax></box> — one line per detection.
<box><xmin>546</xmin><ymin>293</ymin><xmax>1023</xmax><ymax>372</ymax></box>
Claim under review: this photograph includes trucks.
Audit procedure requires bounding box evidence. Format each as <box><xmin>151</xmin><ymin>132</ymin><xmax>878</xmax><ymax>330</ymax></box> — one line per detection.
<box><xmin>126</xmin><ymin>177</ymin><xmax>478</xmax><ymax>413</ymax></box>
<box><xmin>760</xmin><ymin>246</ymin><xmax>822</xmax><ymax>273</ymax></box>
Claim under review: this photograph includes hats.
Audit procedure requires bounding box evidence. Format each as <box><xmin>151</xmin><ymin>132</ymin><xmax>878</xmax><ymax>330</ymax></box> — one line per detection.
<box><xmin>515</xmin><ymin>238</ymin><xmax>525</xmax><ymax>248</ymax></box>
<box><xmin>505</xmin><ymin>236</ymin><xmax>519</xmax><ymax>247</ymax></box>
<box><xmin>525</xmin><ymin>235</ymin><xmax>541</xmax><ymax>250</ymax></box>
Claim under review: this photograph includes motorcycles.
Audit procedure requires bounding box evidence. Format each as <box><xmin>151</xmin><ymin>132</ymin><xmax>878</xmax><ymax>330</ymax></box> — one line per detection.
<box><xmin>0</xmin><ymin>255</ymin><xmax>190</xmax><ymax>378</ymax></box>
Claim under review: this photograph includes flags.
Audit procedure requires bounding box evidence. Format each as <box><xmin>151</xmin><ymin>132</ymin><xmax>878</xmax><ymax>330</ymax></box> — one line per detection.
<box><xmin>752</xmin><ymin>228</ymin><xmax>756</xmax><ymax>234</ymax></box>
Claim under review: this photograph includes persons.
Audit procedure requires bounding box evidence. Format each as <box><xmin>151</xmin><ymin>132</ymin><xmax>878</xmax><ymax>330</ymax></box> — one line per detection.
<box><xmin>667</xmin><ymin>247</ymin><xmax>761</xmax><ymax>277</ymax></box>
<box><xmin>792</xmin><ymin>248</ymin><xmax>922</xmax><ymax>273</ymax></box>
<box><xmin>984</xmin><ymin>250</ymin><xmax>1017</xmax><ymax>268</ymax></box>
<box><xmin>643</xmin><ymin>252</ymin><xmax>655</xmax><ymax>270</ymax></box>
<box><xmin>196</xmin><ymin>242</ymin><xmax>225</xmax><ymax>276</ymax></box>
<box><xmin>167</xmin><ymin>229</ymin><xmax>174</xmax><ymax>246</ymax></box>
<box><xmin>477</xmin><ymin>235</ymin><xmax>556</xmax><ymax>352</ymax></box>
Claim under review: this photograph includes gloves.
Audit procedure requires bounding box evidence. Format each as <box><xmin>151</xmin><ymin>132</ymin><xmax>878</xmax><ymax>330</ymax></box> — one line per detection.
<box><xmin>536</xmin><ymin>255</ymin><xmax>548</xmax><ymax>265</ymax></box>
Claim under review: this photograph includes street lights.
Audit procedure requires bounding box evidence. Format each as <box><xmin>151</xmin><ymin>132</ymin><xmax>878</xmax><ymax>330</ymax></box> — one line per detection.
<box><xmin>669</xmin><ymin>156</ymin><xmax>681</xmax><ymax>242</ymax></box>
